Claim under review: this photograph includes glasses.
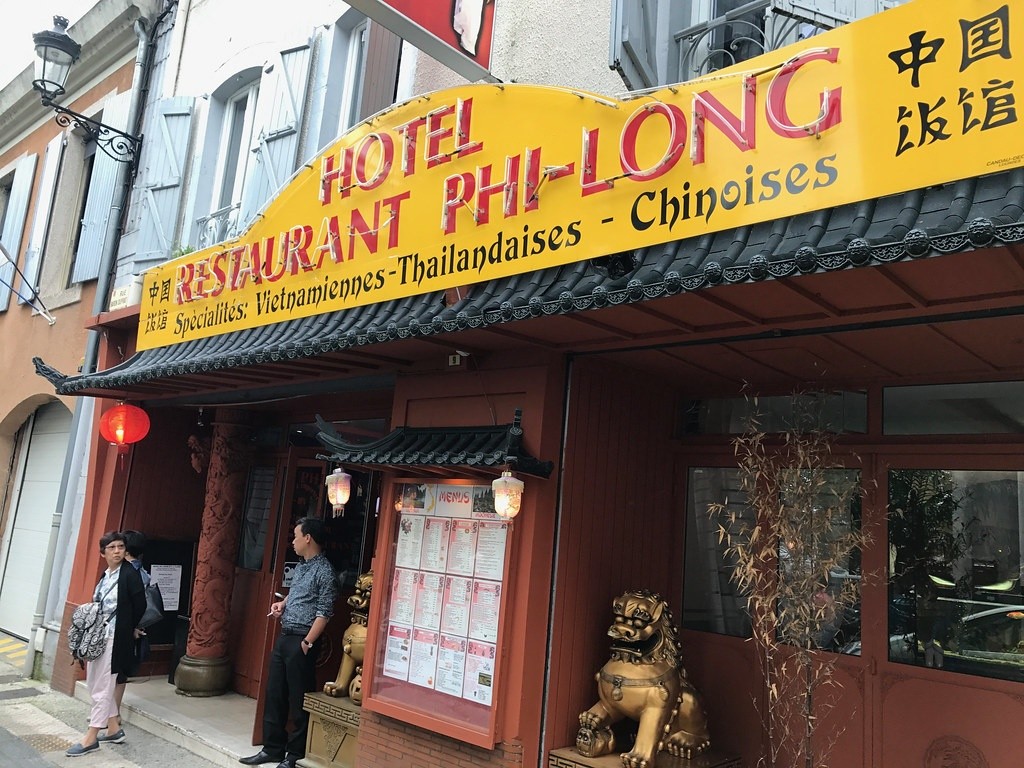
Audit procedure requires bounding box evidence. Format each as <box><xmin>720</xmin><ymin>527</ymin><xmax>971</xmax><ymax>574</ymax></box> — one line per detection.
<box><xmin>104</xmin><ymin>544</ymin><xmax>124</xmax><ymax>550</ymax></box>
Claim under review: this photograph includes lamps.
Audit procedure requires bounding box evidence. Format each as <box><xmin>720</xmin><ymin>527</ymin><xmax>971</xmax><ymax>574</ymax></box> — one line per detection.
<box><xmin>491</xmin><ymin>470</ymin><xmax>524</xmax><ymax>529</ymax></box>
<box><xmin>324</xmin><ymin>467</ymin><xmax>352</xmax><ymax>519</ymax></box>
<box><xmin>32</xmin><ymin>15</ymin><xmax>82</xmax><ymax>107</ymax></box>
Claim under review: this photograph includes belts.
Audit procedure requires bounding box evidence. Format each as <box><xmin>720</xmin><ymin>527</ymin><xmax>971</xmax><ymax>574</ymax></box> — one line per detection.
<box><xmin>280</xmin><ymin>628</ymin><xmax>309</xmax><ymax>635</ymax></box>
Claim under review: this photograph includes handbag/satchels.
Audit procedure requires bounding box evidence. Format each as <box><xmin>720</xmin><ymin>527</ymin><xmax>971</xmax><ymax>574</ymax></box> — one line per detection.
<box><xmin>132</xmin><ymin>583</ymin><xmax>165</xmax><ymax>629</ymax></box>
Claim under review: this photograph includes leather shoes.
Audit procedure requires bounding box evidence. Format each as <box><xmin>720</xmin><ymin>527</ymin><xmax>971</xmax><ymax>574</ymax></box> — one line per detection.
<box><xmin>239</xmin><ymin>752</ymin><xmax>284</xmax><ymax>765</ymax></box>
<box><xmin>275</xmin><ymin>758</ymin><xmax>296</xmax><ymax>768</ymax></box>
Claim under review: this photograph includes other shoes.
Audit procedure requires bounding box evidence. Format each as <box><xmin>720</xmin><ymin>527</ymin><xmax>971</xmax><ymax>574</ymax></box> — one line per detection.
<box><xmin>65</xmin><ymin>740</ymin><xmax>99</xmax><ymax>756</ymax></box>
<box><xmin>97</xmin><ymin>730</ymin><xmax>125</xmax><ymax>744</ymax></box>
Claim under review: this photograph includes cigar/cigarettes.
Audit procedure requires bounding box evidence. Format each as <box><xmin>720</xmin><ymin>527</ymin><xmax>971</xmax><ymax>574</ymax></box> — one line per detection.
<box><xmin>267</xmin><ymin>609</ymin><xmax>278</xmax><ymax>617</ymax></box>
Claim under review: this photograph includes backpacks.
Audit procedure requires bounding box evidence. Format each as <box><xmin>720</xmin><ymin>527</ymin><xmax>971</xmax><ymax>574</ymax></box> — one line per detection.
<box><xmin>67</xmin><ymin>578</ymin><xmax>120</xmax><ymax>662</ymax></box>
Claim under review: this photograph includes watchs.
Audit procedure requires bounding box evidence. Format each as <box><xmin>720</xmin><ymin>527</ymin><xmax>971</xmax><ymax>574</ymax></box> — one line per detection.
<box><xmin>302</xmin><ymin>638</ymin><xmax>313</xmax><ymax>648</ymax></box>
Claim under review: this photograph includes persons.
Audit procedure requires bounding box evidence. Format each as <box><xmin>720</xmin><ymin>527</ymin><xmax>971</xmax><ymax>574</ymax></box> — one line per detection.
<box><xmin>65</xmin><ymin>531</ymin><xmax>127</xmax><ymax>756</ymax></box>
<box><xmin>87</xmin><ymin>529</ymin><xmax>165</xmax><ymax>729</ymax></box>
<box><xmin>239</xmin><ymin>516</ymin><xmax>339</xmax><ymax>768</ymax></box>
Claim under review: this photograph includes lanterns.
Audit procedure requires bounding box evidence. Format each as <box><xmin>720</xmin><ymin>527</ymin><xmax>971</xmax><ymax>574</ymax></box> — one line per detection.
<box><xmin>491</xmin><ymin>471</ymin><xmax>523</xmax><ymax>532</ymax></box>
<box><xmin>100</xmin><ymin>402</ymin><xmax>150</xmax><ymax>471</ymax></box>
<box><xmin>325</xmin><ymin>468</ymin><xmax>351</xmax><ymax>518</ymax></box>
